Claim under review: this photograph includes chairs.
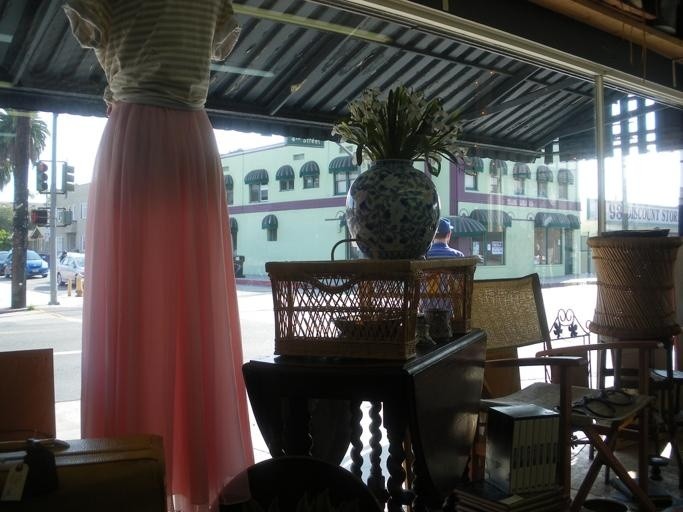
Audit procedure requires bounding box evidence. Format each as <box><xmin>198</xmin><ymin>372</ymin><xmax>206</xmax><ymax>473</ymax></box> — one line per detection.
<box><xmin>532</xmin><ymin>342</ymin><xmax>663</xmax><ymax>512</ymax></box>
<box><xmin>471</xmin><ymin>272</ymin><xmax>665</xmax><ymax>512</ymax></box>
<box><xmin>202</xmin><ymin>461</ymin><xmax>388</xmax><ymax>512</ymax></box>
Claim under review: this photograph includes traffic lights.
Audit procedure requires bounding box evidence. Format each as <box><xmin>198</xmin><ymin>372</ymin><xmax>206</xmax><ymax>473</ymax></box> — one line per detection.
<box><xmin>62</xmin><ymin>164</ymin><xmax>75</xmax><ymax>192</ymax></box>
<box><xmin>36</xmin><ymin>161</ymin><xmax>48</xmax><ymax>192</ymax></box>
<box><xmin>62</xmin><ymin>210</ymin><xmax>72</xmax><ymax>225</ymax></box>
<box><xmin>31</xmin><ymin>209</ymin><xmax>47</xmax><ymax>225</ymax></box>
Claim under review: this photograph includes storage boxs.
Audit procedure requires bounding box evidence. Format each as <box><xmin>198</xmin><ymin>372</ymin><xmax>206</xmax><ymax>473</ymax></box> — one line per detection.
<box><xmin>263</xmin><ymin>264</ymin><xmax>477</xmax><ymax>362</ymax></box>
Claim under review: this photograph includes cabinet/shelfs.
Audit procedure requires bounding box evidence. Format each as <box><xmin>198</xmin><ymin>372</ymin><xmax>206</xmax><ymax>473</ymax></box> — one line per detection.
<box><xmin>245</xmin><ymin>327</ymin><xmax>486</xmax><ymax>512</ymax></box>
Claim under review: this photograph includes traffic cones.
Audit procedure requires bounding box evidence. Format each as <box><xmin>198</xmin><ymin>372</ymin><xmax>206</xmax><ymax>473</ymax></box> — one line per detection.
<box><xmin>75</xmin><ymin>273</ymin><xmax>83</xmax><ymax>297</ymax></box>
<box><xmin>67</xmin><ymin>278</ymin><xmax>72</xmax><ymax>296</ymax></box>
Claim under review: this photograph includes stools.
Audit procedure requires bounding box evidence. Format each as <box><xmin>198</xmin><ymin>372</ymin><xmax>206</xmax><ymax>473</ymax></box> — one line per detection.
<box><xmin>592</xmin><ymin>335</ymin><xmax>664</xmax><ymax>496</ymax></box>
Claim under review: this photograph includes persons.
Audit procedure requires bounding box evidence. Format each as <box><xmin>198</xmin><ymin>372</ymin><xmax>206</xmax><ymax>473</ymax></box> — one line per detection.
<box><xmin>428</xmin><ymin>218</ymin><xmax>464</xmax><ymax>257</ymax></box>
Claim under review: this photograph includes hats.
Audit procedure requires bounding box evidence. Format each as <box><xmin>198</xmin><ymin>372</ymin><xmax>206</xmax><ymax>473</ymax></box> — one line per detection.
<box><xmin>434</xmin><ymin>218</ymin><xmax>454</xmax><ymax>232</ymax></box>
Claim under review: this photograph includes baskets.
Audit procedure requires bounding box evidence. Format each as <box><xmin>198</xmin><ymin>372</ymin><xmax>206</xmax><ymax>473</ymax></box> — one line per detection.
<box><xmin>589</xmin><ymin>235</ymin><xmax>683</xmax><ymax>339</ymax></box>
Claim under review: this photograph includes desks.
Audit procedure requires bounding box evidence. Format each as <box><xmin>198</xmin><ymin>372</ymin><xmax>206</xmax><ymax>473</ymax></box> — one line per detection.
<box><xmin>0</xmin><ymin>436</ymin><xmax>166</xmax><ymax>512</ymax></box>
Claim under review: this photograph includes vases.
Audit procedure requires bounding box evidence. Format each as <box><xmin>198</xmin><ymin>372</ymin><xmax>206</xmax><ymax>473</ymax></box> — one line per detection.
<box><xmin>345</xmin><ymin>159</ymin><xmax>441</xmax><ymax>260</ymax></box>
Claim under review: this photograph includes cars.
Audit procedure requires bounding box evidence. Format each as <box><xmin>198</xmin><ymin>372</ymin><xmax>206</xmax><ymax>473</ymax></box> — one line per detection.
<box><xmin>0</xmin><ymin>251</ymin><xmax>9</xmax><ymax>275</ymax></box>
<box><xmin>3</xmin><ymin>250</ymin><xmax>48</xmax><ymax>277</ymax></box>
<box><xmin>56</xmin><ymin>253</ymin><xmax>85</xmax><ymax>285</ymax></box>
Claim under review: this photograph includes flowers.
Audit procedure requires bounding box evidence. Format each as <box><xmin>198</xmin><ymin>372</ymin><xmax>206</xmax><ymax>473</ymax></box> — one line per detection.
<box><xmin>330</xmin><ymin>85</ymin><xmax>476</xmax><ymax>177</ymax></box>
